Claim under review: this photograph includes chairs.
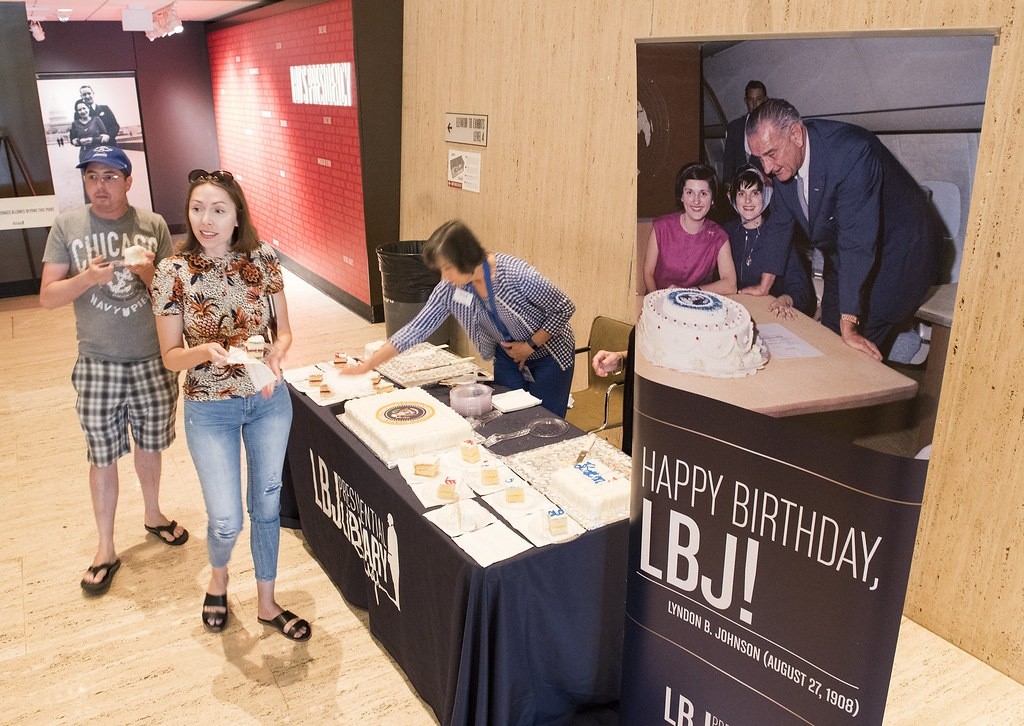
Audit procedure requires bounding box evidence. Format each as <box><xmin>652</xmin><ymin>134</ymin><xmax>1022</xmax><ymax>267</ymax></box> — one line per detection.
<box><xmin>565</xmin><ymin>315</ymin><xmax>635</xmax><ymax>434</ymax></box>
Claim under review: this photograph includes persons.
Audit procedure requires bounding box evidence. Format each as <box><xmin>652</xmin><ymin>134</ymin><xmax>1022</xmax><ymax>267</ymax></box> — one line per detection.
<box><xmin>39</xmin><ymin>146</ymin><xmax>189</xmax><ymax>592</ymax></box>
<box><xmin>73</xmin><ymin>86</ymin><xmax>120</xmax><ymax>148</ymax></box>
<box><xmin>722</xmin><ymin>80</ymin><xmax>772</xmax><ymax>202</ymax></box>
<box><xmin>57</xmin><ymin>137</ymin><xmax>64</xmax><ymax>147</ymax></box>
<box><xmin>70</xmin><ymin>101</ymin><xmax>107</xmax><ymax>205</ymax></box>
<box><xmin>151</xmin><ymin>168</ymin><xmax>313</xmax><ymax>643</ymax></box>
<box><xmin>716</xmin><ymin>165</ymin><xmax>815</xmax><ymax>320</ymax></box>
<box><xmin>738</xmin><ymin>99</ymin><xmax>946</xmax><ymax>362</ymax></box>
<box><xmin>342</xmin><ymin>220</ymin><xmax>576</xmax><ymax>417</ymax></box>
<box><xmin>592</xmin><ymin>161</ymin><xmax>737</xmax><ymax>378</ymax></box>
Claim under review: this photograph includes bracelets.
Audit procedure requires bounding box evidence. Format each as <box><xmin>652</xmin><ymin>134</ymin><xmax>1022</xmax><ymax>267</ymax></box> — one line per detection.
<box><xmin>841</xmin><ymin>314</ymin><xmax>859</xmax><ymax>326</ymax></box>
<box><xmin>696</xmin><ymin>286</ymin><xmax>702</xmax><ymax>290</ymax></box>
<box><xmin>611</xmin><ymin>352</ymin><xmax>624</xmax><ymax>375</ymax></box>
<box><xmin>527</xmin><ymin>338</ymin><xmax>538</xmax><ymax>351</ymax></box>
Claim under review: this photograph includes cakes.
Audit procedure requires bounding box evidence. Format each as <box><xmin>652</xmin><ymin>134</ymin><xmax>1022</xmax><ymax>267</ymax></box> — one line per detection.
<box><xmin>307</xmin><ymin>370</ymin><xmax>331</xmax><ymax>396</ymax></box>
<box><xmin>371</xmin><ymin>372</ymin><xmax>394</xmax><ymax>394</ymax></box>
<box><xmin>462</xmin><ymin>440</ymin><xmax>480</xmax><ymax>464</ymax></box>
<box><xmin>415</xmin><ymin>454</ymin><xmax>441</xmax><ymax>477</ymax></box>
<box><xmin>437</xmin><ymin>474</ymin><xmax>460</xmax><ymax>500</ymax></box>
<box><xmin>245</xmin><ymin>335</ymin><xmax>265</xmax><ymax>358</ymax></box>
<box><xmin>482</xmin><ymin>461</ymin><xmax>501</xmax><ymax>485</ymax></box>
<box><xmin>638</xmin><ymin>288</ymin><xmax>761</xmax><ymax>374</ymax></box>
<box><xmin>344</xmin><ymin>386</ymin><xmax>471</xmax><ymax>459</ymax></box>
<box><xmin>547</xmin><ymin>508</ymin><xmax>568</xmax><ymax>536</ymax></box>
<box><xmin>335</xmin><ymin>351</ymin><xmax>348</xmax><ymax>367</ymax></box>
<box><xmin>506</xmin><ymin>482</ymin><xmax>525</xmax><ymax>502</ymax></box>
<box><xmin>551</xmin><ymin>458</ymin><xmax>631</xmax><ymax>520</ymax></box>
<box><xmin>123</xmin><ymin>245</ymin><xmax>146</xmax><ymax>265</ymax></box>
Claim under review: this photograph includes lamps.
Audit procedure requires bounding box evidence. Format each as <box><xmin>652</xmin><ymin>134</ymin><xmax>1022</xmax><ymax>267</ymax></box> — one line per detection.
<box><xmin>145</xmin><ymin>7</ymin><xmax>184</xmax><ymax>42</ymax></box>
<box><xmin>29</xmin><ymin>20</ymin><xmax>45</xmax><ymax>41</ymax></box>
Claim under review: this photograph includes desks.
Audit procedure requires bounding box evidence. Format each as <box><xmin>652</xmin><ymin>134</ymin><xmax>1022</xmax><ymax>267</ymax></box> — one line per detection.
<box><xmin>636</xmin><ymin>293</ymin><xmax>919</xmax><ymax>419</ymax></box>
<box><xmin>279</xmin><ymin>342</ymin><xmax>633</xmax><ymax>726</ymax></box>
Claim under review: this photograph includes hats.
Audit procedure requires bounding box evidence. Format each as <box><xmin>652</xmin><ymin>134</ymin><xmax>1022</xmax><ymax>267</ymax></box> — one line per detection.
<box><xmin>75</xmin><ymin>146</ymin><xmax>133</xmax><ymax>176</ymax></box>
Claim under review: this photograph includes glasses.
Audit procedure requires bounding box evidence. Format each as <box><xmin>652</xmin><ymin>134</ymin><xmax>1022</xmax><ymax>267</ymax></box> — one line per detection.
<box><xmin>78</xmin><ymin>172</ymin><xmax>126</xmax><ymax>184</ymax></box>
<box><xmin>187</xmin><ymin>169</ymin><xmax>234</xmax><ymax>186</ymax></box>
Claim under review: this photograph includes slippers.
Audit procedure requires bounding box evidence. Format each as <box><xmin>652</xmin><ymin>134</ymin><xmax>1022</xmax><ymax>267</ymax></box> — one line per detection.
<box><xmin>144</xmin><ymin>518</ymin><xmax>189</xmax><ymax>546</ymax></box>
<box><xmin>257</xmin><ymin>608</ymin><xmax>312</xmax><ymax>642</ymax></box>
<box><xmin>202</xmin><ymin>572</ymin><xmax>230</xmax><ymax>633</ymax></box>
<box><xmin>80</xmin><ymin>557</ymin><xmax>120</xmax><ymax>592</ymax></box>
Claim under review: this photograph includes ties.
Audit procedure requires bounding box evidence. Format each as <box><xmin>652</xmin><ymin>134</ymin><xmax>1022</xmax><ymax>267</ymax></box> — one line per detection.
<box><xmin>796</xmin><ymin>173</ymin><xmax>808</xmax><ymax>220</ymax></box>
